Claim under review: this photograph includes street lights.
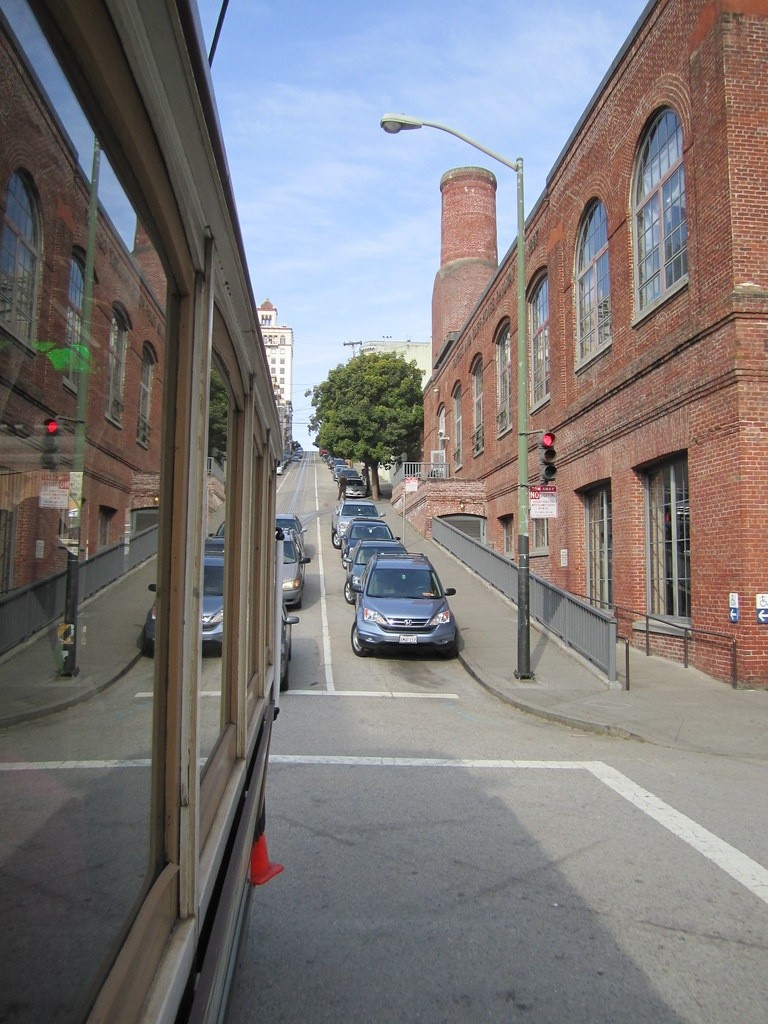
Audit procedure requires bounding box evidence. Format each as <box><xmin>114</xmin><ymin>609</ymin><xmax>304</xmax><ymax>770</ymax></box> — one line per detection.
<box><xmin>379</xmin><ymin>110</ymin><xmax>535</xmax><ymax>681</ymax></box>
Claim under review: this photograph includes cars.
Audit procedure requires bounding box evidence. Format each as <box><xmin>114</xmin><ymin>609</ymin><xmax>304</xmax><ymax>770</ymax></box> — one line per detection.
<box><xmin>350</xmin><ymin>550</ymin><xmax>459</xmax><ymax>657</ymax></box>
<box><xmin>318</xmin><ymin>444</ymin><xmax>370</xmax><ymax>498</ymax></box>
<box><xmin>344</xmin><ymin>537</ymin><xmax>408</xmax><ymax>603</ymax></box>
<box><xmin>340</xmin><ymin>516</ymin><xmax>396</xmax><ymax>569</ymax></box>
<box><xmin>274</xmin><ymin>514</ymin><xmax>305</xmax><ymax>548</ymax></box>
<box><xmin>140</xmin><ymin>516</ymin><xmax>229</xmax><ymax>650</ymax></box>
<box><xmin>331</xmin><ymin>500</ymin><xmax>381</xmax><ymax>550</ymax></box>
<box><xmin>279</xmin><ymin>602</ymin><xmax>301</xmax><ymax>692</ymax></box>
<box><xmin>277</xmin><ymin>438</ymin><xmax>303</xmax><ymax>475</ymax></box>
<box><xmin>271</xmin><ymin>526</ymin><xmax>312</xmax><ymax>609</ymax></box>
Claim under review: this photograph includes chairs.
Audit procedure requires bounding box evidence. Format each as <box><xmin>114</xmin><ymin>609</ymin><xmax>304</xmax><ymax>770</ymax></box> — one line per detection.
<box><xmin>374</xmin><ymin>573</ymin><xmax>395</xmax><ymax>595</ymax></box>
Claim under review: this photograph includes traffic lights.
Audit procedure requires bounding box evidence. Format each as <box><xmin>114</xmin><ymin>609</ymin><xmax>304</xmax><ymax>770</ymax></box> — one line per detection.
<box><xmin>539</xmin><ymin>432</ymin><xmax>558</xmax><ymax>482</ymax></box>
<box><xmin>38</xmin><ymin>415</ymin><xmax>63</xmax><ymax>471</ymax></box>
